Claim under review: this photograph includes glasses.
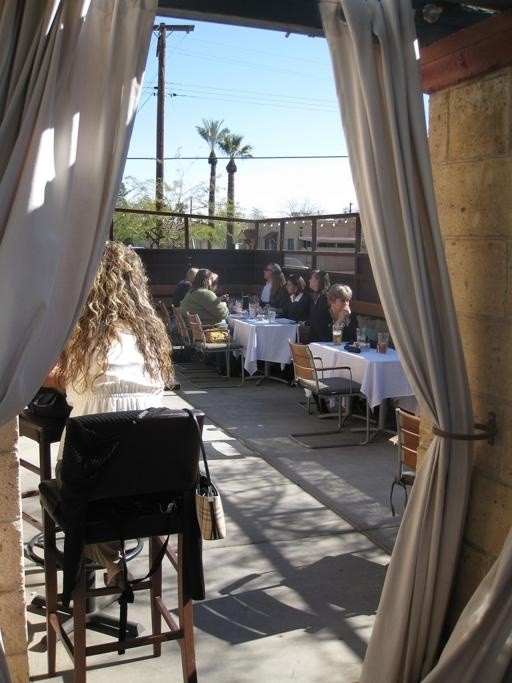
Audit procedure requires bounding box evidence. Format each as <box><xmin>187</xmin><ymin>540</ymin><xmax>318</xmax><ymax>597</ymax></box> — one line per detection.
<box><xmin>265</xmin><ymin>267</ymin><xmax>273</xmax><ymax>271</ymax></box>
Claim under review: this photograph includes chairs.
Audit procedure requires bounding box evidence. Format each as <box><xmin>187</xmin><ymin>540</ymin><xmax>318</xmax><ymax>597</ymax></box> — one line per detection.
<box><xmin>389</xmin><ymin>408</ymin><xmax>421</xmax><ymax>517</ymax></box>
<box><xmin>288</xmin><ymin>341</ymin><xmax>370</xmax><ymax>449</ymax></box>
<box><xmin>39</xmin><ymin>409</ymin><xmax>206</xmax><ymax>683</ymax></box>
<box><xmin>295</xmin><ymin>326</ymin><xmax>316</xmax><ymax>416</ymax></box>
<box><xmin>159</xmin><ymin>300</ymin><xmax>244</xmax><ymax>389</ymax></box>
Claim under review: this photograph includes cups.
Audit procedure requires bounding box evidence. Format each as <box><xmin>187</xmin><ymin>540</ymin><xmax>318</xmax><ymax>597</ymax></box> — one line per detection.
<box><xmin>236</xmin><ymin>299</ymin><xmax>243</xmax><ymax>315</ymax></box>
<box><xmin>269</xmin><ymin>310</ymin><xmax>276</xmax><ymax>322</ymax></box>
<box><xmin>356</xmin><ymin>327</ymin><xmax>366</xmax><ymax>342</ymax></box>
<box><xmin>377</xmin><ymin>332</ymin><xmax>390</xmax><ymax>352</ymax></box>
<box><xmin>333</xmin><ymin>323</ymin><xmax>343</xmax><ymax>345</ymax></box>
<box><xmin>249</xmin><ymin>303</ymin><xmax>256</xmax><ymax>318</ymax></box>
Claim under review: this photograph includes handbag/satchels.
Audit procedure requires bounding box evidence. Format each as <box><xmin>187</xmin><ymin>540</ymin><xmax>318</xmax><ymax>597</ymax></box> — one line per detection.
<box><xmin>205</xmin><ymin>327</ymin><xmax>232</xmax><ymax>343</ymax></box>
<box><xmin>195</xmin><ymin>475</ymin><xmax>227</xmax><ymax>539</ymax></box>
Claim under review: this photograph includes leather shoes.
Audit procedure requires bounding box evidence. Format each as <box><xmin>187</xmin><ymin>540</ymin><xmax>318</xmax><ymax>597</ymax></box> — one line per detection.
<box><xmin>103</xmin><ymin>570</ymin><xmax>126</xmax><ymax>591</ymax></box>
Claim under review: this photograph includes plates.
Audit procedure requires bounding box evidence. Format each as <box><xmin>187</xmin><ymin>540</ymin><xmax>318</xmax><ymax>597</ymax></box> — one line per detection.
<box><xmin>277</xmin><ymin>319</ymin><xmax>296</xmax><ymax>323</ymax></box>
<box><xmin>230</xmin><ymin>313</ymin><xmax>247</xmax><ymax>318</ymax></box>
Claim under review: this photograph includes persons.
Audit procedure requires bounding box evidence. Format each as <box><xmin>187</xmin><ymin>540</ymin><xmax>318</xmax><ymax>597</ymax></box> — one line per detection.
<box><xmin>259</xmin><ymin>261</ymin><xmax>281</xmax><ymax>305</ymax></box>
<box><xmin>285</xmin><ymin>273</ymin><xmax>304</xmax><ymax>305</ymax></box>
<box><xmin>269</xmin><ymin>271</ymin><xmax>286</xmax><ymax>307</ymax></box>
<box><xmin>307</xmin><ymin>268</ymin><xmax>331</xmax><ymax>301</ymax></box>
<box><xmin>175</xmin><ymin>266</ymin><xmax>198</xmax><ymax>307</ymax></box>
<box><xmin>41</xmin><ymin>237</ymin><xmax>176</xmax><ymax>587</ymax></box>
<box><xmin>180</xmin><ymin>270</ymin><xmax>230</xmax><ymax>340</ymax></box>
<box><xmin>308</xmin><ymin>282</ymin><xmax>363</xmax><ymax>343</ymax></box>
